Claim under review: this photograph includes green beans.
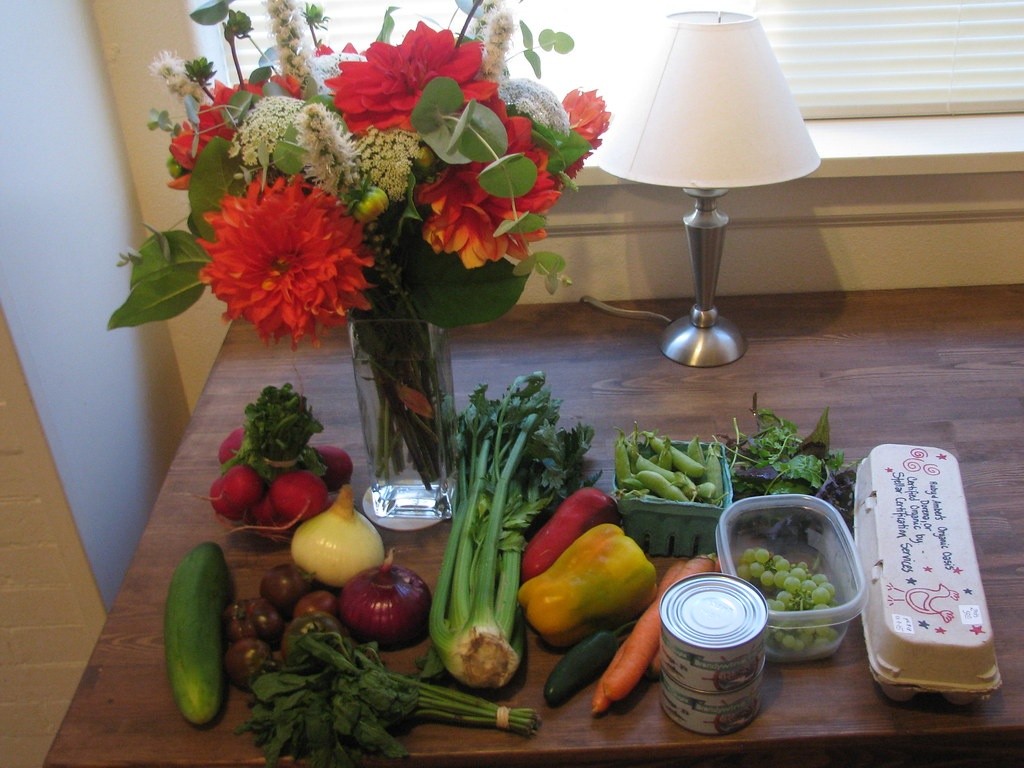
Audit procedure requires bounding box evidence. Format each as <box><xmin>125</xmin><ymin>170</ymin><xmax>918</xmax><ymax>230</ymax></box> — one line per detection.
<box><xmin>613</xmin><ymin>418</ymin><xmax>727</xmax><ymax>507</ymax></box>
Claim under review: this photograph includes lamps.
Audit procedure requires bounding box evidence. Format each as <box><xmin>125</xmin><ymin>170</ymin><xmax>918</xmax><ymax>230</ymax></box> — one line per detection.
<box><xmin>591</xmin><ymin>10</ymin><xmax>823</xmax><ymax>368</ymax></box>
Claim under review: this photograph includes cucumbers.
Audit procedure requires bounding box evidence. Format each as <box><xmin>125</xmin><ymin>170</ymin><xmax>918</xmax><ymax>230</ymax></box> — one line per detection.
<box><xmin>162</xmin><ymin>541</ymin><xmax>226</xmax><ymax>724</ymax></box>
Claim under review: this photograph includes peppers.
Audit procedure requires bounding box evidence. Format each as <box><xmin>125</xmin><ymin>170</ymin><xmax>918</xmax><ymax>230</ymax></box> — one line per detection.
<box><xmin>517</xmin><ymin>489</ymin><xmax>658</xmax><ymax>707</ymax></box>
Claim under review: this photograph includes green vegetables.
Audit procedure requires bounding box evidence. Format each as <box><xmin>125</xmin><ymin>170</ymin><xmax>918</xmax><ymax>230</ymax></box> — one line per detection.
<box><xmin>711</xmin><ymin>393</ymin><xmax>865</xmax><ymax>537</ymax></box>
<box><xmin>232</xmin><ymin>626</ymin><xmax>540</xmax><ymax>768</ymax></box>
<box><xmin>428</xmin><ymin>369</ymin><xmax>606</xmax><ymax>691</ymax></box>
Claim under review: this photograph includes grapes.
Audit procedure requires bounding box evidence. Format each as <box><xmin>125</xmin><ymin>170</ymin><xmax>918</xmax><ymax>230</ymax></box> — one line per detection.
<box><xmin>736</xmin><ymin>546</ymin><xmax>842</xmax><ymax>657</ymax></box>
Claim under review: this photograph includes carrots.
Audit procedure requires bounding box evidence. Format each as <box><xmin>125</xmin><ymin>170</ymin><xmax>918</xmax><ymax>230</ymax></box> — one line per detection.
<box><xmin>590</xmin><ymin>552</ymin><xmax>722</xmax><ymax>719</ymax></box>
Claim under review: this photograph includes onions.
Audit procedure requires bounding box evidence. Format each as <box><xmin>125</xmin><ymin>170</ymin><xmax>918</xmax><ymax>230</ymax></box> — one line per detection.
<box><xmin>226</xmin><ymin>484</ymin><xmax>432</xmax><ymax>692</ymax></box>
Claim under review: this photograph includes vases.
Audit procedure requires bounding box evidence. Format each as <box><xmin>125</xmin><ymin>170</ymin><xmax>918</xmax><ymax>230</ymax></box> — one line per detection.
<box><xmin>347</xmin><ymin>320</ymin><xmax>461</xmax><ymax>534</ymax></box>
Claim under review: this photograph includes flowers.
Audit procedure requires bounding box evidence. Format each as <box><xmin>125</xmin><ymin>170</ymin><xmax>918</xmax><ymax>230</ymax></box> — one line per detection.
<box><xmin>105</xmin><ymin>2</ymin><xmax>612</xmax><ymax>351</ymax></box>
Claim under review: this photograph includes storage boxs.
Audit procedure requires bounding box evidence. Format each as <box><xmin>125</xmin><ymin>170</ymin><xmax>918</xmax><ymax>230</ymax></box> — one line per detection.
<box><xmin>853</xmin><ymin>443</ymin><xmax>1003</xmax><ymax>705</ymax></box>
<box><xmin>611</xmin><ymin>438</ymin><xmax>735</xmax><ymax>559</ymax></box>
<box><xmin>714</xmin><ymin>493</ymin><xmax>870</xmax><ymax>665</ymax></box>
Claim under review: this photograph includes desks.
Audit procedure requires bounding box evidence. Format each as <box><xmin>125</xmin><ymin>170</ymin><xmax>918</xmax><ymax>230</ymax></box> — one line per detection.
<box><xmin>38</xmin><ymin>280</ymin><xmax>1023</xmax><ymax>768</ymax></box>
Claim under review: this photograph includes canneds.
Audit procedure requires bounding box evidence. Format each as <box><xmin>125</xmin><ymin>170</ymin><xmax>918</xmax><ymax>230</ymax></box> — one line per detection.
<box><xmin>659</xmin><ymin>571</ymin><xmax>769</xmax><ymax>736</ymax></box>
